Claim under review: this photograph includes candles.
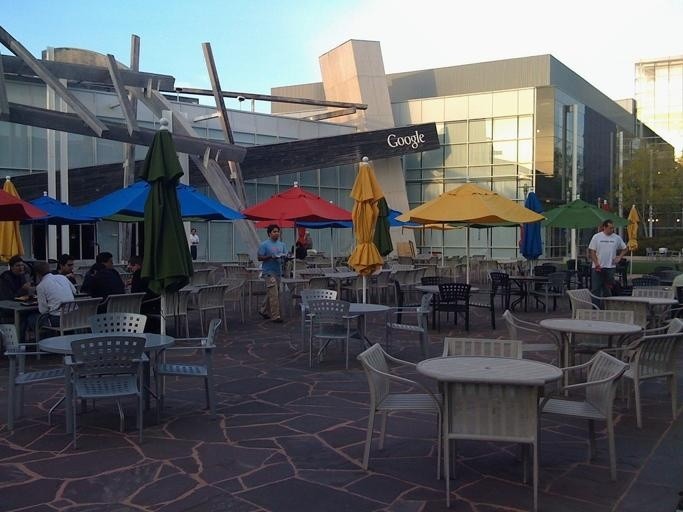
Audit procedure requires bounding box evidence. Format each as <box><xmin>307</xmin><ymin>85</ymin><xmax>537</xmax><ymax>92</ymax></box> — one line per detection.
<box><xmin>249</xmin><ymin>276</ymin><xmax>309</xmax><ymax>319</ymax></box>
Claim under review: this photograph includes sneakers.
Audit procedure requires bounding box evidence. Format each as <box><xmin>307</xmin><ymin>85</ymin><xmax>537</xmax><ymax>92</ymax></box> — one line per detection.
<box><xmin>259</xmin><ymin>311</ymin><xmax>270</xmax><ymax>319</ymax></box>
<box><xmin>272</xmin><ymin>317</ymin><xmax>283</xmax><ymax>324</ymax></box>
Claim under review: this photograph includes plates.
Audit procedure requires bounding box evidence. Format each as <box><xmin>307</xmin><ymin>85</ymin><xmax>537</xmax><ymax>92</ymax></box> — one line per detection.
<box><xmin>13</xmin><ymin>296</ymin><xmax>31</xmax><ymax>303</ymax></box>
<box><xmin>21</xmin><ymin>301</ymin><xmax>38</xmax><ymax>306</ymax></box>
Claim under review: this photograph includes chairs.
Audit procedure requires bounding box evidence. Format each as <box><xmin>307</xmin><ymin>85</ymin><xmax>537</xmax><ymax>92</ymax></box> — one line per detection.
<box><xmin>437</xmin><ymin>375</ymin><xmax>545</xmax><ymax>511</ymax></box>
<box><xmin>537</xmin><ymin>351</ymin><xmax>626</xmax><ymax>482</ymax></box>
<box><xmin>181</xmin><ymin>280</ymin><xmax>211</xmax><ymax>333</ymax></box>
<box><xmin>300</xmin><ymin>288</ymin><xmax>338</xmax><ymax>352</ymax></box>
<box><xmin>357</xmin><ymin>342</ymin><xmax>443</xmax><ymax>481</ymax></box>
<box><xmin>1</xmin><ymin>324</ymin><xmax>66</xmax><ymax>432</ymax></box>
<box><xmin>568</xmin><ymin>307</ymin><xmax>634</xmax><ymax>399</ymax></box>
<box><xmin>631</xmin><ymin>285</ymin><xmax>676</xmax><ymax>327</ymax></box>
<box><xmin>589</xmin><ymin>333</ymin><xmax>683</xmax><ymax>429</ymax></box>
<box><xmin>490</xmin><ymin>272</ymin><xmax>510</xmax><ymax>310</ymax></box>
<box><xmin>34</xmin><ymin>298</ymin><xmax>102</xmax><ymax>360</ymax></box>
<box><xmin>442</xmin><ymin>339</ymin><xmax>525</xmax><ymax>361</ymax></box>
<box><xmin>67</xmin><ymin>336</ymin><xmax>149</xmax><ymax>447</ymax></box>
<box><xmin>141</xmin><ymin>289</ymin><xmax>191</xmax><ymax>344</ymax></box>
<box><xmin>567</xmin><ymin>288</ymin><xmax>600</xmax><ymax>311</ymax></box>
<box><xmin>502</xmin><ymin>309</ymin><xmax>563</xmax><ymax>399</ymax></box>
<box><xmin>99</xmin><ymin>292</ymin><xmax>145</xmax><ymax>332</ymax></box>
<box><xmin>405</xmin><ymin>268</ymin><xmax>428</xmax><ymax>302</ymax></box>
<box><xmin>602</xmin><ymin>298</ymin><xmax>649</xmax><ymax>355</ymax></box>
<box><xmin>671</xmin><ymin>275</ymin><xmax>683</xmax><ymax>320</ymax></box>
<box><xmin>621</xmin><ymin>319</ymin><xmax>683</xmax><ymax>408</ymax></box>
<box><xmin>421</xmin><ymin>275</ymin><xmax>458</xmax><ymax>324</ymax></box>
<box><xmin>88</xmin><ymin>312</ymin><xmax>146</xmax><ymax>410</ymax></box>
<box><xmin>341</xmin><ymin>266</ymin><xmax>390</xmax><ymax>305</ymax></box>
<box><xmin>216</xmin><ymin>277</ymin><xmax>246</xmax><ymax>327</ymax></box>
<box><xmin>531</xmin><ymin>271</ymin><xmax>565</xmax><ymax>310</ymax></box>
<box><xmin>187</xmin><ymin>284</ymin><xmax>229</xmax><ymax>336</ymax></box>
<box><xmin>238</xmin><ymin>253</ymin><xmax>254</xmax><ymax>273</ymax></box>
<box><xmin>306</xmin><ymin>299</ymin><xmax>362</xmax><ymax>369</ymax></box>
<box><xmin>153</xmin><ymin>317</ymin><xmax>222</xmax><ymax>416</ymax></box>
<box><xmin>534</xmin><ymin>265</ymin><xmax>554</xmax><ymax>308</ymax></box>
<box><xmin>433</xmin><ymin>283</ymin><xmax>469</xmax><ymax>333</ymax></box>
<box><xmin>385</xmin><ymin>270</ymin><xmax>408</xmax><ymax>304</ymax></box>
<box><xmin>644</xmin><ymin>247</ymin><xmax>682</xmax><ymax>264</ymax></box>
<box><xmin>394</xmin><ymin>280</ymin><xmax>430</xmax><ymax>328</ymax></box>
<box><xmin>431</xmin><ymin>252</ymin><xmax>528</xmax><ymax>283</ymax></box>
<box><xmin>385</xmin><ymin>293</ymin><xmax>432</xmax><ymax>361</ymax></box>
<box><xmin>631</xmin><ymin>276</ymin><xmax>659</xmax><ymax>286</ymax></box>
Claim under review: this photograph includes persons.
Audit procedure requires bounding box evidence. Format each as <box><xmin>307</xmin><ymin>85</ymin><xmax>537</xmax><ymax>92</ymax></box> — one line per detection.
<box><xmin>24</xmin><ymin>262</ymin><xmax>78</xmax><ymax>351</ymax></box>
<box><xmin>288</xmin><ymin>227</ymin><xmax>313</xmax><ymax>260</ymax></box>
<box><xmin>128</xmin><ymin>225</ymin><xmax>161</xmax><ymax>334</ymax></box>
<box><xmin>256</xmin><ymin>223</ymin><xmax>289</xmax><ymax>323</ymax></box>
<box><xmin>51</xmin><ymin>253</ymin><xmax>79</xmax><ymax>291</ymax></box>
<box><xmin>79</xmin><ymin>252</ymin><xmax>126</xmax><ymax>313</ymax></box>
<box><xmin>588</xmin><ymin>219</ymin><xmax>629</xmax><ymax>310</ymax></box>
<box><xmin>188</xmin><ymin>228</ymin><xmax>199</xmax><ymax>260</ymax></box>
<box><xmin>0</xmin><ymin>255</ymin><xmax>31</xmax><ymax>300</ymax></box>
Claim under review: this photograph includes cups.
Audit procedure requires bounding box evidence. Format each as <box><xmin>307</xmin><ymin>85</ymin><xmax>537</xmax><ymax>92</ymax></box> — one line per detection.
<box><xmin>28</xmin><ymin>287</ymin><xmax>35</xmax><ymax>300</ymax></box>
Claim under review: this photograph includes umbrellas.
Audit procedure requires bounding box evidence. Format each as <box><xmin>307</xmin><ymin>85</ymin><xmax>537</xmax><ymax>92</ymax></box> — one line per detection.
<box><xmin>627</xmin><ymin>204</ymin><xmax>641</xmax><ymax>286</ymax></box>
<box><xmin>519</xmin><ymin>188</ymin><xmax>544</xmax><ymax>277</ymax></box>
<box><xmin>0</xmin><ymin>175</ymin><xmax>25</xmax><ymax>270</ymax></box>
<box><xmin>78</xmin><ymin>178</ymin><xmax>249</xmax><ymax>221</ymax></box>
<box><xmin>101</xmin><ymin>212</ymin><xmax>213</xmax><ymax>223</ymax></box>
<box><xmin>445</xmin><ymin>221</ymin><xmax>520</xmax><ymax>293</ymax></box>
<box><xmin>517</xmin><ymin>222</ymin><xmax>523</xmax><ymax>247</ymax></box>
<box><xmin>253</xmin><ymin>219</ymin><xmax>295</xmax><ymax>228</ymax></box>
<box><xmin>240</xmin><ymin>181</ymin><xmax>352</xmax><ymax>221</ymax></box>
<box><xmin>19</xmin><ymin>190</ymin><xmax>99</xmax><ymax>226</ymax></box>
<box><xmin>537</xmin><ymin>194</ymin><xmax>636</xmax><ymax>289</ymax></box>
<box><xmin>392</xmin><ymin>177</ymin><xmax>549</xmax><ymax>304</ymax></box>
<box><xmin>346</xmin><ymin>164</ymin><xmax>386</xmax><ymax>343</ymax></box>
<box><xmin>387</xmin><ymin>206</ymin><xmax>423</xmax><ymax>226</ymax></box>
<box><xmin>136</xmin><ymin>117</ymin><xmax>195</xmax><ymax>399</ymax></box>
<box><xmin>0</xmin><ymin>188</ymin><xmax>49</xmax><ymax>222</ymax></box>
<box><xmin>599</xmin><ymin>199</ymin><xmax>612</xmax><ymax>236</ymax></box>
<box><xmin>293</xmin><ymin>219</ymin><xmax>353</xmax><ymax>228</ymax></box>
<box><xmin>373</xmin><ymin>196</ymin><xmax>393</xmax><ymax>258</ymax></box>
<box><xmin>399</xmin><ymin>223</ymin><xmax>464</xmax><ymax>266</ymax></box>
